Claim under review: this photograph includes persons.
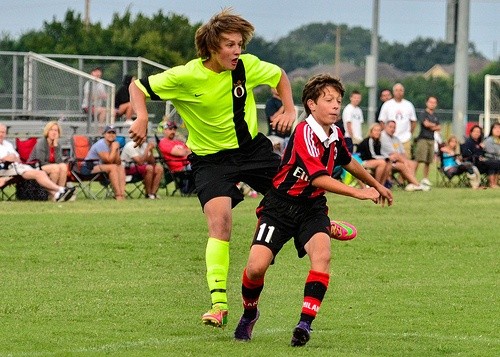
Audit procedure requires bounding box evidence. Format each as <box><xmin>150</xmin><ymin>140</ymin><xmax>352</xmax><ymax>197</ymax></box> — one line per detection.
<box><xmin>0</xmin><ymin>121</ymin><xmax>195</xmax><ymax>202</ymax></box>
<box><xmin>129</xmin><ymin>9</ymin><xmax>357</xmax><ymax>329</ymax></box>
<box><xmin>238</xmin><ymin>82</ymin><xmax>500</xmax><ymax>198</ymax></box>
<box><xmin>82</xmin><ymin>66</ymin><xmax>107</xmax><ymax>126</ymax></box>
<box><xmin>115</xmin><ymin>75</ymin><xmax>137</xmax><ymax>122</ymax></box>
<box><xmin>235</xmin><ymin>72</ymin><xmax>393</xmax><ymax>346</ymax></box>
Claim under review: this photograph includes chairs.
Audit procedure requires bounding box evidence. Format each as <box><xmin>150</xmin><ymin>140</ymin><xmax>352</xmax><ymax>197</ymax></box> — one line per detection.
<box><xmin>1</xmin><ymin>137</ymin><xmax>193</xmax><ymax>199</ymax></box>
<box><xmin>435</xmin><ymin>142</ymin><xmax>489</xmax><ymax>186</ymax></box>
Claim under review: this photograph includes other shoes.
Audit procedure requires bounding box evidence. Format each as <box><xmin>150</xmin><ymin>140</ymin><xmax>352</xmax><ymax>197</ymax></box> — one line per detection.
<box><xmin>55</xmin><ymin>186</ymin><xmax>77</xmax><ymax>202</ymax></box>
<box><xmin>404</xmin><ymin>183</ymin><xmax>430</xmax><ymax>191</ymax></box>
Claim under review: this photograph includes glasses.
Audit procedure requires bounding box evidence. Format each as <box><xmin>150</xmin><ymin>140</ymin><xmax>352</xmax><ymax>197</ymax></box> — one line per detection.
<box><xmin>169</xmin><ymin>127</ymin><xmax>177</xmax><ymax>130</ymax></box>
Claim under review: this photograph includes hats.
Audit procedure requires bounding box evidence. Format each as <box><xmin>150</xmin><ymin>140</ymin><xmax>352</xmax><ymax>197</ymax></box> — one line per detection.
<box><xmin>163</xmin><ymin>122</ymin><xmax>178</xmax><ymax>130</ymax></box>
<box><xmin>102</xmin><ymin>126</ymin><xmax>116</xmax><ymax>134</ymax></box>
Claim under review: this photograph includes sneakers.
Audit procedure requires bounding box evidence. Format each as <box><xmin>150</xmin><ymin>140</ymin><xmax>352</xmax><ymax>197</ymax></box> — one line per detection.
<box><xmin>234</xmin><ymin>310</ymin><xmax>260</xmax><ymax>340</ymax></box>
<box><xmin>328</xmin><ymin>220</ymin><xmax>357</xmax><ymax>240</ymax></box>
<box><xmin>291</xmin><ymin>321</ymin><xmax>310</xmax><ymax>346</ymax></box>
<box><xmin>203</xmin><ymin>309</ymin><xmax>229</xmax><ymax>329</ymax></box>
